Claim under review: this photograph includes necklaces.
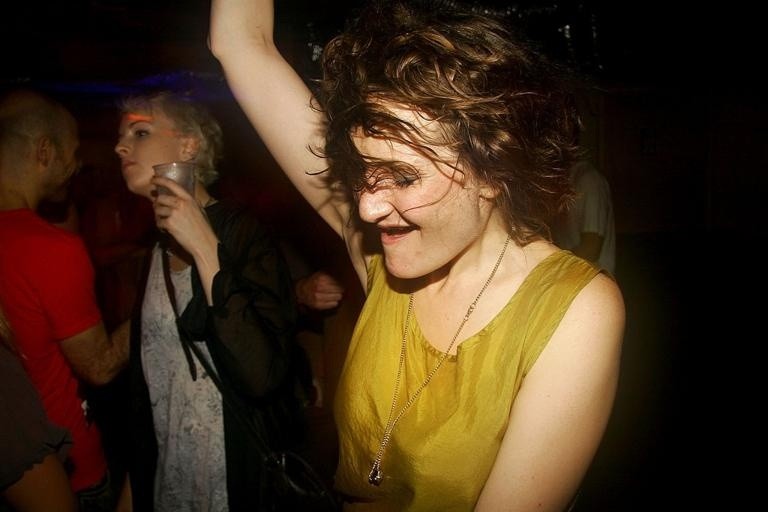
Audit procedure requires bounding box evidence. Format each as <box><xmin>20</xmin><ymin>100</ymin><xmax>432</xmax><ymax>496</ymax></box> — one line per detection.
<box><xmin>368</xmin><ymin>225</ymin><xmax>514</xmax><ymax>484</ymax></box>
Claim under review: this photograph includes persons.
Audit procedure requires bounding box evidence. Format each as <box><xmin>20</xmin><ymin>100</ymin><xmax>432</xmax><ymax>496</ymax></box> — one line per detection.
<box><xmin>210</xmin><ymin>0</ymin><xmax>629</xmax><ymax>510</ymax></box>
<box><xmin>554</xmin><ymin>165</ymin><xmax>614</xmax><ymax>275</ymax></box>
<box><xmin>2</xmin><ymin>89</ymin><xmax>347</xmax><ymax>509</ymax></box>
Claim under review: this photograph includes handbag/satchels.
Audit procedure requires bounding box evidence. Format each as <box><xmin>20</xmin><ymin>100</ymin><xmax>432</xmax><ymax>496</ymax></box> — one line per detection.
<box><xmin>266</xmin><ymin>439</ymin><xmax>337</xmax><ymax>509</ymax></box>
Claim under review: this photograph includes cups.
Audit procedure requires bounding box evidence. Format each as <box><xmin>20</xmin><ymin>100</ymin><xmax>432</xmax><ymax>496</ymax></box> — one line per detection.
<box><xmin>152</xmin><ymin>161</ymin><xmax>196</xmax><ymax>227</ymax></box>
